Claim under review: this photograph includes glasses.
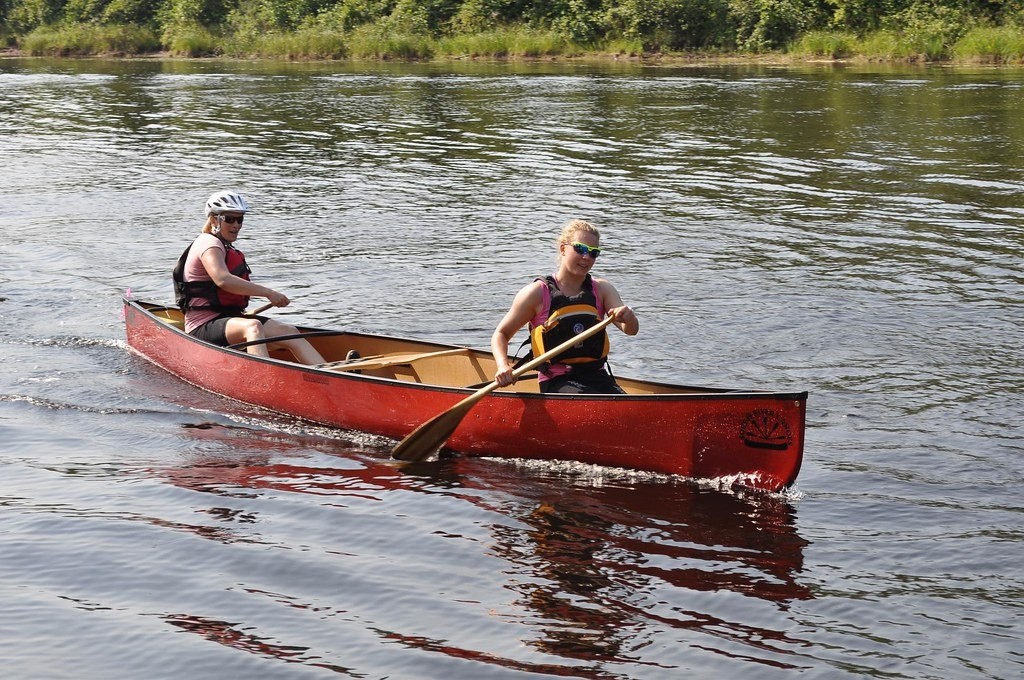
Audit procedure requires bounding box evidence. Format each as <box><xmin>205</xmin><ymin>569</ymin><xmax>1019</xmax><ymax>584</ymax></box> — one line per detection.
<box><xmin>214</xmin><ymin>215</ymin><xmax>244</xmax><ymax>224</ymax></box>
<box><xmin>563</xmin><ymin>241</ymin><xmax>601</xmax><ymax>257</ymax></box>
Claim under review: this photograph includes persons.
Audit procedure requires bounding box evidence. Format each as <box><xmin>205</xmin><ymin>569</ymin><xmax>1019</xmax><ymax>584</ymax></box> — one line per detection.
<box><xmin>173</xmin><ymin>188</ymin><xmax>362</xmax><ymax>375</ymax></box>
<box><xmin>490</xmin><ymin>220</ymin><xmax>640</xmax><ymax>394</ymax></box>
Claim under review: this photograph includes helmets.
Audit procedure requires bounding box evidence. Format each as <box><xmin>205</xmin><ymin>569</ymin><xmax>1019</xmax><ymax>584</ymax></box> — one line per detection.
<box><xmin>205</xmin><ymin>190</ymin><xmax>251</xmax><ymax>218</ymax></box>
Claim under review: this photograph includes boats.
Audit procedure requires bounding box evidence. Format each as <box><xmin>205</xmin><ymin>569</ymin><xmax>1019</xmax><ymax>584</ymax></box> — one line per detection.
<box><xmin>122</xmin><ymin>296</ymin><xmax>808</xmax><ymax>494</ymax></box>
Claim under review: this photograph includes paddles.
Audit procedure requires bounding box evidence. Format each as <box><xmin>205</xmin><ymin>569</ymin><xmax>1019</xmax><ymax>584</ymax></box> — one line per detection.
<box><xmin>249</xmin><ymin>302</ymin><xmax>274</xmax><ymax>315</ymax></box>
<box><xmin>391</xmin><ymin>317</ymin><xmax>614</xmax><ymax>463</ymax></box>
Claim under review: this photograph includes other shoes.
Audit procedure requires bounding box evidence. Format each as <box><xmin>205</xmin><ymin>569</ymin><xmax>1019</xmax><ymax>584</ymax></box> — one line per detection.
<box><xmin>344</xmin><ymin>349</ymin><xmax>362</xmax><ymax>374</ymax></box>
